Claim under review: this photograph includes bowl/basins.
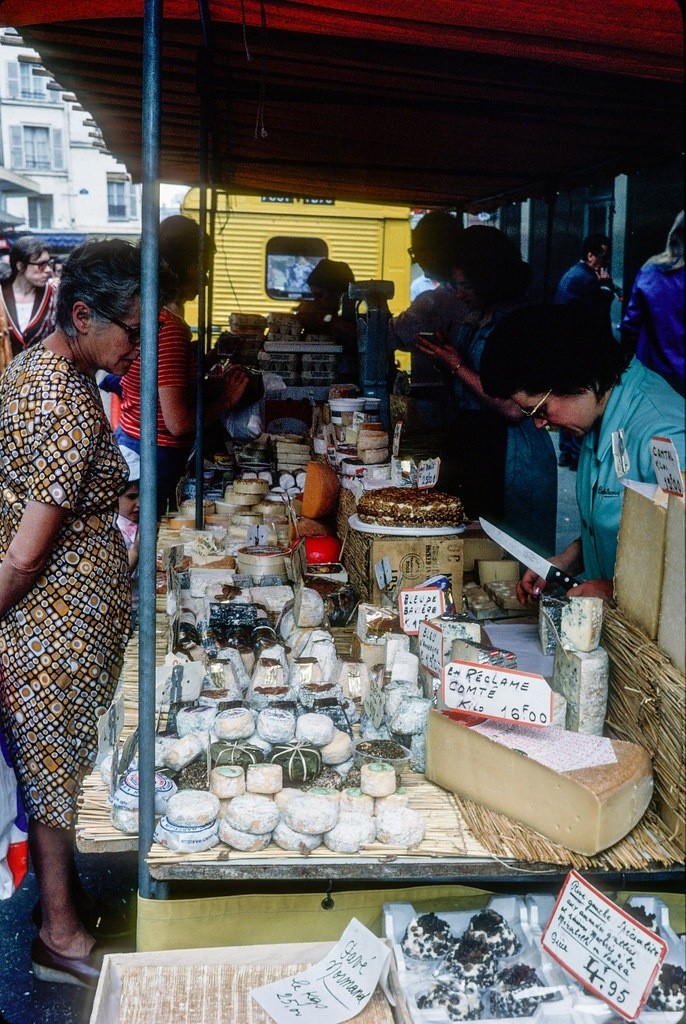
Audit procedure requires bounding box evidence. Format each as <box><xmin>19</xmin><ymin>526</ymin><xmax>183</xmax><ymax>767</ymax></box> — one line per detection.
<box><xmin>348</xmin><ymin>740</ymin><xmax>415</xmax><ymax>776</ymax></box>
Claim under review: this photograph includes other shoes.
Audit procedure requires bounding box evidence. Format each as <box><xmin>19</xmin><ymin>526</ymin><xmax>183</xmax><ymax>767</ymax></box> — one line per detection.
<box><xmin>31</xmin><ymin>897</ymin><xmax>134</xmax><ymax>936</ymax></box>
<box><xmin>31</xmin><ymin>926</ymin><xmax>131</xmax><ymax>987</ymax></box>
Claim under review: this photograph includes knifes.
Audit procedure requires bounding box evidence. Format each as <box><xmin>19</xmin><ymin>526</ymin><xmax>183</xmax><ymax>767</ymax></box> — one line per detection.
<box><xmin>479</xmin><ymin>517</ymin><xmax>583</xmax><ymax>588</ymax></box>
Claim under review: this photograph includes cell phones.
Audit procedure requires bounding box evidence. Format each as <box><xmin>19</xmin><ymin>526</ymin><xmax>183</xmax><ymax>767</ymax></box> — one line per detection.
<box><xmin>419</xmin><ymin>333</ymin><xmax>444</xmax><ymax>348</ymax></box>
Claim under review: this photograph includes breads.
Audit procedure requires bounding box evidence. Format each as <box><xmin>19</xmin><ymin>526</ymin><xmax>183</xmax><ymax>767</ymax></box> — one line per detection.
<box><xmin>97</xmin><ymin>442</ymin><xmax>433</xmax><ymax>852</ymax></box>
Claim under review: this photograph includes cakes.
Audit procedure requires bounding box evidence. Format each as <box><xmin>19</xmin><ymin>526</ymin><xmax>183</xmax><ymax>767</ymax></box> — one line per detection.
<box><xmin>355</xmin><ymin>487</ymin><xmax>463</xmax><ymax>528</ymax></box>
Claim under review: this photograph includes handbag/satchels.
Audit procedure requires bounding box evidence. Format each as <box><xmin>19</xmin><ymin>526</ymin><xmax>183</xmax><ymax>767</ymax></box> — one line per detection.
<box><xmin>0</xmin><ymin>730</ymin><xmax>30</xmax><ymax>900</ymax></box>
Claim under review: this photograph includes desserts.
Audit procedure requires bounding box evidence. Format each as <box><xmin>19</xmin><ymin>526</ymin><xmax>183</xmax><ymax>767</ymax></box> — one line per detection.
<box><xmin>400</xmin><ymin>903</ymin><xmax>686</xmax><ymax>1024</ymax></box>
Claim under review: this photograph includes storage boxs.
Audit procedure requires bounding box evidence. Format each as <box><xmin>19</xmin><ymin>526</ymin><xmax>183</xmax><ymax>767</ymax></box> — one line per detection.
<box><xmin>228</xmin><ymin>300</ymin><xmax>339</xmax><ymax>383</ymax></box>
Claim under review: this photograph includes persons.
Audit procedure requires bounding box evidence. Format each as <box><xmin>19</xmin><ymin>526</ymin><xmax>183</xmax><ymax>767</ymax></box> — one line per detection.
<box><xmin>292</xmin><ymin>210</ymin><xmax>471</xmax><ymax>426</ymax></box>
<box><xmin>0</xmin><ymin>237</ymin><xmax>165</xmax><ymax>989</ymax></box>
<box><xmin>0</xmin><ymin>236</ymin><xmax>60</xmax><ymax>358</ymax></box>
<box><xmin>558</xmin><ymin>234</ymin><xmax>615</xmax><ymax>471</ymax></box>
<box><xmin>416</xmin><ymin>225</ymin><xmax>557</xmax><ymax>557</ymax></box>
<box><xmin>478</xmin><ymin>302</ymin><xmax>686</xmax><ymax>605</ymax></box>
<box><xmin>116</xmin><ymin>445</ymin><xmax>139</xmax><ymax>612</ymax></box>
<box><xmin>114</xmin><ymin>213</ymin><xmax>250</xmax><ymax>515</ymax></box>
<box><xmin>614</xmin><ymin>206</ymin><xmax>686</xmax><ymax>392</ymax></box>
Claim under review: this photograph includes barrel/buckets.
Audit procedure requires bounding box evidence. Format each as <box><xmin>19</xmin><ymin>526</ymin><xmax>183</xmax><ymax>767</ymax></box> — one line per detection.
<box><xmin>329</xmin><ymin>398</ymin><xmax>366</xmax><ymax>427</ymax></box>
<box><xmin>357</xmin><ymin>397</ymin><xmax>381</xmax><ymax>422</ymax></box>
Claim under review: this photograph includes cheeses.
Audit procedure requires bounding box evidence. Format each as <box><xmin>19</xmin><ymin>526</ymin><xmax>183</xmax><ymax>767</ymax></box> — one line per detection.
<box><xmin>334</xmin><ymin>411</ymin><xmax>388</xmax><ymax>464</ymax></box>
<box><xmin>422</xmin><ymin>527</ymin><xmax>655</xmax><ymax>857</ymax></box>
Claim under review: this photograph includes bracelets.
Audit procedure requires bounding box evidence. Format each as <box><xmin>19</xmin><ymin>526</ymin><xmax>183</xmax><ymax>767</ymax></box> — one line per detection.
<box><xmin>451</xmin><ymin>362</ymin><xmax>464</xmax><ymax>376</ymax></box>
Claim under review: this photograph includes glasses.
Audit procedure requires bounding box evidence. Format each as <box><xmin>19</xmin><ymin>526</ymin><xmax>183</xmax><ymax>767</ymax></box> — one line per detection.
<box><xmin>407</xmin><ymin>246</ymin><xmax>426</xmax><ymax>265</ymax></box>
<box><xmin>91</xmin><ymin>308</ymin><xmax>165</xmax><ymax>344</ymax></box>
<box><xmin>26</xmin><ymin>259</ymin><xmax>55</xmax><ymax>271</ymax></box>
<box><xmin>519</xmin><ymin>387</ymin><xmax>553</xmax><ymax>417</ymax></box>
